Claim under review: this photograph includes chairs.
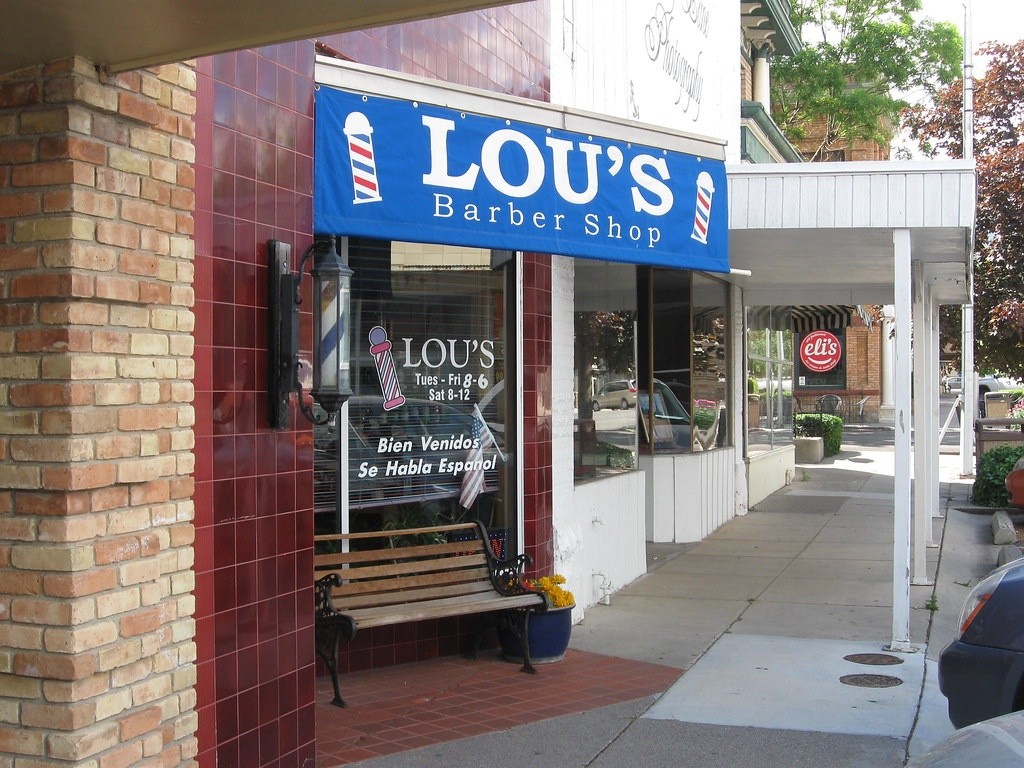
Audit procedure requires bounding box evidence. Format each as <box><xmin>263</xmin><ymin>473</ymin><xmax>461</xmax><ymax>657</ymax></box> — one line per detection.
<box><xmin>815</xmin><ymin>394</ymin><xmax>842</xmax><ymax>415</ymax></box>
<box><xmin>840</xmin><ymin>395</ymin><xmax>870</xmax><ymax>427</ymax></box>
<box><xmin>792</xmin><ymin>394</ymin><xmax>818</xmax><ymax>414</ymax></box>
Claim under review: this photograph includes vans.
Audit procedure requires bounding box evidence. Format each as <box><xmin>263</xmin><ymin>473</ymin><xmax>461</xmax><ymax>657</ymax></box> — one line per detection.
<box><xmin>591</xmin><ymin>379</ymin><xmax>639</xmax><ymax>412</ymax></box>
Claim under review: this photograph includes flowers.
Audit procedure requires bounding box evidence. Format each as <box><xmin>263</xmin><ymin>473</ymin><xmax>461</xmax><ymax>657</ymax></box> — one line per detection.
<box><xmin>505</xmin><ymin>575</ymin><xmax>575</xmax><ymax>608</ymax></box>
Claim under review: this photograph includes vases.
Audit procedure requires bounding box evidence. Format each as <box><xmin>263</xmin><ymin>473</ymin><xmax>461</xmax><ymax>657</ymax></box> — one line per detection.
<box><xmin>496</xmin><ymin>604</ymin><xmax>577</xmax><ymax>663</ymax></box>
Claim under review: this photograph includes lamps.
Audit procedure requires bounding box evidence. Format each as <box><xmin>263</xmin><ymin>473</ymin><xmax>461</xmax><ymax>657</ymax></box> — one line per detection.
<box><xmin>266</xmin><ymin>232</ymin><xmax>355</xmax><ymax>431</ymax></box>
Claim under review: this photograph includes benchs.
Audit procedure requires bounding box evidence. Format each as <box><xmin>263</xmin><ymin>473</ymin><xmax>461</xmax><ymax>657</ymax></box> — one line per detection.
<box><xmin>314</xmin><ymin>521</ymin><xmax>550</xmax><ymax>708</ymax></box>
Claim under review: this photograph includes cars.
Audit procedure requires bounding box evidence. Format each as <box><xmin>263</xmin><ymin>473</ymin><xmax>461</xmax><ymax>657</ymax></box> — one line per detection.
<box><xmin>903</xmin><ymin>556</ymin><xmax>1024</xmax><ymax>768</ymax></box>
<box><xmin>313</xmin><ymin>395</ymin><xmax>510</xmax><ymax>543</ymax></box>
<box><xmin>941</xmin><ymin>373</ymin><xmax>1021</xmax><ymax>419</ymax></box>
